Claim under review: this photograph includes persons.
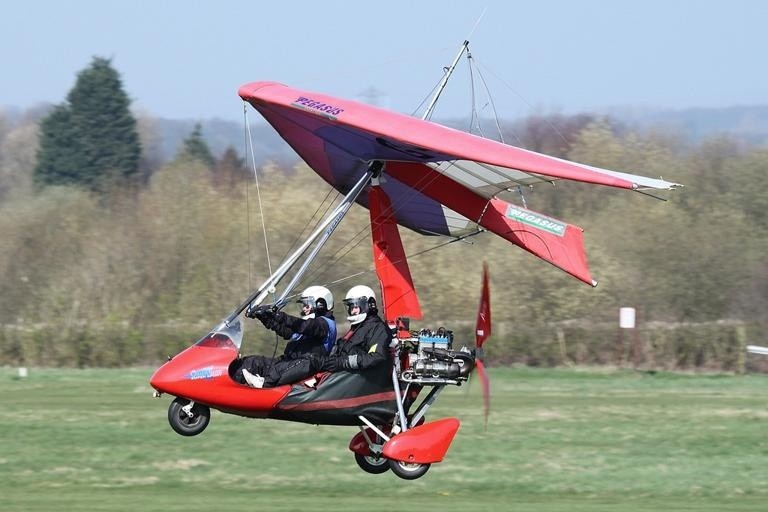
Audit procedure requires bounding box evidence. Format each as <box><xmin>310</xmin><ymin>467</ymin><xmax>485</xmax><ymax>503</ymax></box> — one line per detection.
<box><xmin>242</xmin><ymin>283</ymin><xmax>392</xmax><ymax>390</ymax></box>
<box><xmin>229</xmin><ymin>284</ymin><xmax>338</xmax><ymax>384</ymax></box>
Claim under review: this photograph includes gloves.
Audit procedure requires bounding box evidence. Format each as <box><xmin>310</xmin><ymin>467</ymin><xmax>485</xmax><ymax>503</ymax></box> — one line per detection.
<box><xmin>325</xmin><ymin>357</ymin><xmax>345</xmax><ymax>372</ymax></box>
<box><xmin>248</xmin><ymin>305</ymin><xmax>284</xmax><ymax>329</ymax></box>
<box><xmin>310</xmin><ymin>351</ymin><xmax>325</xmax><ymax>370</ymax></box>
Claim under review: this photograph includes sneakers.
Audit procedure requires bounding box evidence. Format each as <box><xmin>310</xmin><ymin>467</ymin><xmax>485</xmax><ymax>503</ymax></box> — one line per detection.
<box><xmin>242</xmin><ymin>368</ymin><xmax>265</xmax><ymax>389</ymax></box>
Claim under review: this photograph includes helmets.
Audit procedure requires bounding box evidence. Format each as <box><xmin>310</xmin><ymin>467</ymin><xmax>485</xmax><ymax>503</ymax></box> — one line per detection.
<box><xmin>296</xmin><ymin>285</ymin><xmax>334</xmax><ymax>314</ymax></box>
<box><xmin>344</xmin><ymin>285</ymin><xmax>377</xmax><ymax>325</ymax></box>
